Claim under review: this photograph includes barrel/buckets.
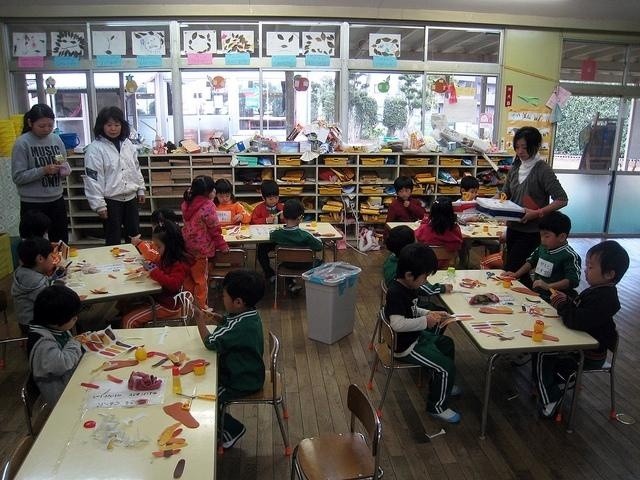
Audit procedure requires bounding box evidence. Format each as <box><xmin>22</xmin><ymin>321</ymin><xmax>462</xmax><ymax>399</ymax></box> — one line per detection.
<box><xmin>59</xmin><ymin>133</ymin><xmax>80</xmax><ymax>156</ymax></box>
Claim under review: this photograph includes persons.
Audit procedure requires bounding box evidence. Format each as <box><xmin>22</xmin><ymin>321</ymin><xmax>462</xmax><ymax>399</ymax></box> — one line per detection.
<box><xmin>10</xmin><ymin>207</ymin><xmax>193</xmax><ymax>336</ymax></box>
<box><xmin>191</xmin><ymin>268</ymin><xmax>265</xmax><ymax>450</ymax></box>
<box><xmin>491</xmin><ymin>125</ymin><xmax>629</xmax><ymax>425</ymax></box>
<box><xmin>10</xmin><ymin>103</ymin><xmax>72</xmax><ymax>248</ymax></box>
<box><xmin>80</xmin><ymin>106</ymin><xmax>145</xmax><ymax>248</ymax></box>
<box><xmin>383</xmin><ymin>176</ymin><xmax>483</xmax><ymax>423</ymax></box>
<box><xmin>27</xmin><ymin>284</ymin><xmax>86</xmax><ymax>411</ymax></box>
<box><xmin>180</xmin><ymin>175</ymin><xmax>324</xmax><ymax>303</ymax></box>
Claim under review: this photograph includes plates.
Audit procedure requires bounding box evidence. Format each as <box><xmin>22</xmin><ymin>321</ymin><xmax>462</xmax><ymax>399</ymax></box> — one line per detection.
<box><xmin>402</xmin><ymin>150</ymin><xmax>421</xmax><ymax>153</ymax></box>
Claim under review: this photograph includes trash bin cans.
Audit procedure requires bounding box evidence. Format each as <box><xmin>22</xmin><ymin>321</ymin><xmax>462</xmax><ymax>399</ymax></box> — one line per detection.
<box><xmin>301</xmin><ymin>262</ymin><xmax>362</xmax><ymax>345</ymax></box>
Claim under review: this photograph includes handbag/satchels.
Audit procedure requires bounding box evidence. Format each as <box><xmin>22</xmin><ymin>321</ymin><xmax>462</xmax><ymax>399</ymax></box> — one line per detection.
<box><xmin>318</xmin><ymin>167</ymin><xmax>339</xmax><ymax>183</ymax></box>
<box><xmin>334</xmin><ymin>224</ymin><xmax>347</xmax><ymax>249</ymax></box>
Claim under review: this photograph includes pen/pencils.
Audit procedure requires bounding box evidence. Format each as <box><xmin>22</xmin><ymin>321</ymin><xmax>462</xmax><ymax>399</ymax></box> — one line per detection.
<box><xmin>65</xmin><ymin>261</ymin><xmax>72</xmax><ymax>268</ymax></box>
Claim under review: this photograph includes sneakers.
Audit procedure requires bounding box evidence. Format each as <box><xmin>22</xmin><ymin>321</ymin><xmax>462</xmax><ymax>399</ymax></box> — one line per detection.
<box><xmin>512</xmin><ymin>351</ymin><xmax>533</xmax><ymax>366</ymax></box>
<box><xmin>269</xmin><ymin>275</ymin><xmax>276</xmax><ymax>283</ymax></box>
<box><xmin>222</xmin><ymin>429</ymin><xmax>246</xmax><ymax>449</ymax></box>
<box><xmin>447</xmin><ymin>384</ymin><xmax>463</xmax><ymax>399</ymax></box>
<box><xmin>426</xmin><ymin>407</ymin><xmax>461</xmax><ymax>423</ymax></box>
<box><xmin>542</xmin><ymin>396</ymin><xmax>562</xmax><ymax>417</ymax></box>
<box><xmin>210</xmin><ymin>280</ymin><xmax>219</xmax><ymax>288</ymax></box>
<box><xmin>557</xmin><ymin>380</ymin><xmax>576</xmax><ymax>390</ymax></box>
<box><xmin>288</xmin><ymin>279</ymin><xmax>303</xmax><ymax>294</ymax></box>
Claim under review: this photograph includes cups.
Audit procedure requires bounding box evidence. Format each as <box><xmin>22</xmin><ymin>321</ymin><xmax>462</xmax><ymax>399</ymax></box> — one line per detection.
<box><xmin>134</xmin><ymin>348</ymin><xmax>146</xmax><ymax>360</ymax></box>
<box><xmin>449</xmin><ymin>141</ymin><xmax>457</xmax><ymax>151</ymax></box>
<box><xmin>310</xmin><ymin>221</ymin><xmax>317</xmax><ymax>227</ymax></box>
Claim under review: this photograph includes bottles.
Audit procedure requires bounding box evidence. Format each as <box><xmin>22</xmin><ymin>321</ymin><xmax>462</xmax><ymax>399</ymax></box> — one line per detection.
<box><xmin>483</xmin><ymin>225</ymin><xmax>489</xmax><ymax>232</ymax></box>
<box><xmin>172</xmin><ymin>367</ymin><xmax>181</xmax><ymax>395</ymax></box>
<box><xmin>192</xmin><ymin>358</ymin><xmax>207</xmax><ymax>377</ymax></box>
<box><xmin>446</xmin><ymin>267</ymin><xmax>456</xmax><ymax>289</ymax></box>
<box><xmin>531</xmin><ymin>320</ymin><xmax>545</xmax><ymax>342</ymax></box>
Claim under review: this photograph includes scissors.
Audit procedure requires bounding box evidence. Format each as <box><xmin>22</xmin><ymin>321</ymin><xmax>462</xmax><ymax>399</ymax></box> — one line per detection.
<box><xmin>500</xmin><ymin>193</ymin><xmax>507</xmax><ymax>203</ymax></box>
<box><xmin>74</xmin><ymin>335</ymin><xmax>103</xmax><ymax>344</ymax></box>
<box><xmin>486</xmin><ymin>272</ymin><xmax>495</xmax><ymax>280</ymax></box>
<box><xmin>462</xmin><ymin>192</ymin><xmax>471</xmax><ymax>201</ymax></box>
<box><xmin>176</xmin><ymin>386</ymin><xmax>216</xmax><ymax>410</ymax></box>
<box><xmin>193</xmin><ymin>302</ymin><xmax>214</xmax><ymax>321</ymax></box>
<box><xmin>430</xmin><ymin>312</ymin><xmax>456</xmax><ymax>321</ymax></box>
